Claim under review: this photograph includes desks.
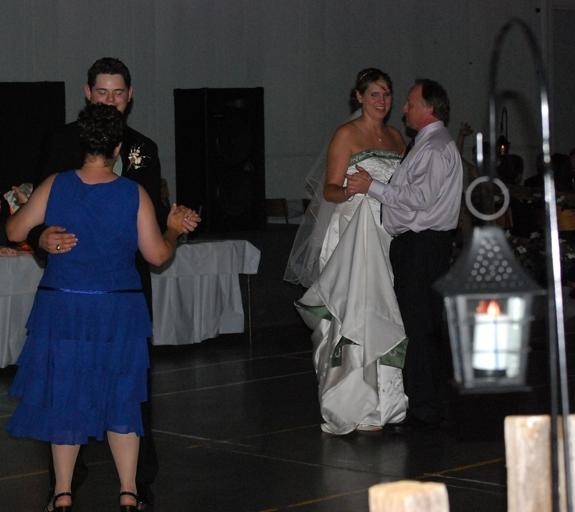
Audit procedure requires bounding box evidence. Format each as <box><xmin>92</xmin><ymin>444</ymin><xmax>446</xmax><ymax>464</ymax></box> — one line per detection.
<box><xmin>0</xmin><ymin>236</ymin><xmax>264</xmax><ymax>371</ymax></box>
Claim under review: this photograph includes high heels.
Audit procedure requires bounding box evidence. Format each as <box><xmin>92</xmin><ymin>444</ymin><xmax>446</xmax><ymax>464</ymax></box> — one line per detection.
<box><xmin>41</xmin><ymin>492</ymin><xmax>73</xmax><ymax>512</ymax></box>
<box><xmin>118</xmin><ymin>492</ymin><xmax>148</xmax><ymax>512</ymax></box>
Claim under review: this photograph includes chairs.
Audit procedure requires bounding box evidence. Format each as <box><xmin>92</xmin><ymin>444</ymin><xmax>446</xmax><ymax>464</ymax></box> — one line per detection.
<box><xmin>502</xmin><ymin>413</ymin><xmax>575</xmax><ymax>512</ymax></box>
<box><xmin>367</xmin><ymin>477</ymin><xmax>452</xmax><ymax>512</ymax></box>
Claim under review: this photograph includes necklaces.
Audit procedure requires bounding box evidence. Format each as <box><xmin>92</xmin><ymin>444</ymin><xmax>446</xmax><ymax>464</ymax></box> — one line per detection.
<box><xmin>364</xmin><ymin>122</ymin><xmax>389</xmax><ymax>144</ymax></box>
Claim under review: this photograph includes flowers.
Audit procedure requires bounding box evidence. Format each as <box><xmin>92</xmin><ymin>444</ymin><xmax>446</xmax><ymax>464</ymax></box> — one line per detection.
<box><xmin>126</xmin><ymin>141</ymin><xmax>152</xmax><ymax>175</ymax></box>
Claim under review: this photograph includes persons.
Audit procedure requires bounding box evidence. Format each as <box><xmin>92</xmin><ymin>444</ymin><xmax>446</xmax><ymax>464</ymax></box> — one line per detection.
<box><xmin>283</xmin><ymin>68</ymin><xmax>413</xmax><ymax>436</ymax></box>
<box><xmin>344</xmin><ymin>81</ymin><xmax>462</xmax><ymax>436</ymax></box>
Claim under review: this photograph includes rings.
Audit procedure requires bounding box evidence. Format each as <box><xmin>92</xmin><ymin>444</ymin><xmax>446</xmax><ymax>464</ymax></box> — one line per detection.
<box><xmin>56</xmin><ymin>244</ymin><xmax>61</xmax><ymax>250</ymax></box>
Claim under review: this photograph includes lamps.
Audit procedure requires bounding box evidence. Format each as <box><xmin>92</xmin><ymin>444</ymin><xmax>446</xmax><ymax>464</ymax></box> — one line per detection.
<box><xmin>430</xmin><ymin>15</ymin><xmax>575</xmax><ymax>512</ymax></box>
